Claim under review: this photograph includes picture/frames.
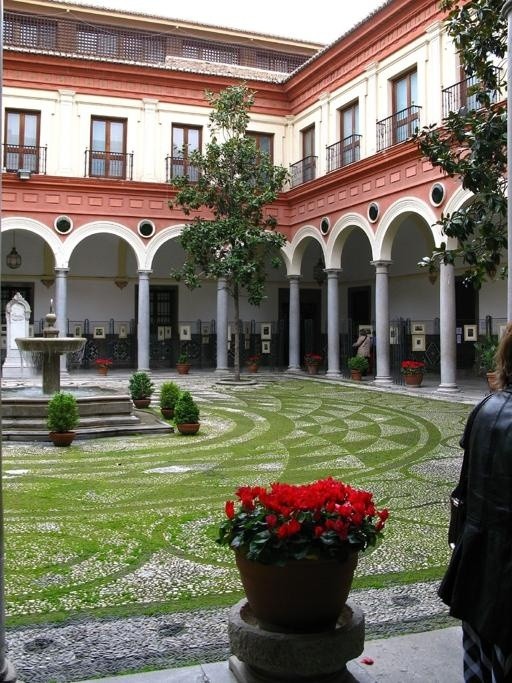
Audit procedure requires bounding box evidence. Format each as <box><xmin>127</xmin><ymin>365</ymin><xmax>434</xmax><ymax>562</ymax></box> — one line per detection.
<box><xmin>68</xmin><ymin>322</ymin><xmax>274</xmax><ymax>354</ymax></box>
<box><xmin>356</xmin><ymin>321</ymin><xmax>508</xmax><ymax>351</ymax></box>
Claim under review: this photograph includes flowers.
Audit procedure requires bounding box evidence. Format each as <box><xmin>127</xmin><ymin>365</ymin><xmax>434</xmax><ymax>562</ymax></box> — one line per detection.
<box><xmin>96</xmin><ymin>357</ymin><xmax>115</xmax><ymax>368</ymax></box>
<box><xmin>399</xmin><ymin>358</ymin><xmax>425</xmax><ymax>375</ymax></box>
<box><xmin>303</xmin><ymin>352</ymin><xmax>321</xmax><ymax>362</ymax></box>
<box><xmin>216</xmin><ymin>478</ymin><xmax>387</xmax><ymax>562</ymax></box>
<box><xmin>246</xmin><ymin>354</ymin><xmax>259</xmax><ymax>364</ymax></box>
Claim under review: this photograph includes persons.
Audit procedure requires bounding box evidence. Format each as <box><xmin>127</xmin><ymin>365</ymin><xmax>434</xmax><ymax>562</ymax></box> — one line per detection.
<box><xmin>437</xmin><ymin>321</ymin><xmax>512</xmax><ymax>683</ymax></box>
<box><xmin>351</xmin><ymin>329</ymin><xmax>372</xmax><ymax>376</ymax></box>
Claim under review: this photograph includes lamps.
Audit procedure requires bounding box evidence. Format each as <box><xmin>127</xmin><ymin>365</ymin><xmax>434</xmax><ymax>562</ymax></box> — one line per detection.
<box><xmin>40</xmin><ymin>279</ymin><xmax>56</xmax><ymax>288</ymax></box>
<box><xmin>17</xmin><ymin>167</ymin><xmax>32</xmax><ymax>183</ymax></box>
<box><xmin>4</xmin><ymin>230</ymin><xmax>24</xmax><ymax>270</ymax></box>
<box><xmin>114</xmin><ymin>280</ymin><xmax>130</xmax><ymax>292</ymax></box>
<box><xmin>312</xmin><ymin>246</ymin><xmax>327</xmax><ymax>287</ymax></box>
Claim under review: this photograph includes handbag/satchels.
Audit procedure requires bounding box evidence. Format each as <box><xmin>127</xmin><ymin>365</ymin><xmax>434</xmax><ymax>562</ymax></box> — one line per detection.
<box><xmin>351</xmin><ymin>345</ymin><xmax>358</xmax><ymax>357</ymax></box>
<box><xmin>449</xmin><ymin>393</ymin><xmax>496</xmax><ymax>550</ymax></box>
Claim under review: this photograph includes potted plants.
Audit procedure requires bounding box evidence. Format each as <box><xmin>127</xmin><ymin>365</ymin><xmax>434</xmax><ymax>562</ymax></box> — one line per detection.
<box><xmin>171</xmin><ymin>389</ymin><xmax>202</xmax><ymax>434</ymax></box>
<box><xmin>480</xmin><ymin>338</ymin><xmax>497</xmax><ymax>389</ymax></box>
<box><xmin>347</xmin><ymin>355</ymin><xmax>368</xmax><ymax>379</ymax></box>
<box><xmin>46</xmin><ymin>391</ymin><xmax>78</xmax><ymax>446</ymax></box>
<box><xmin>129</xmin><ymin>370</ymin><xmax>156</xmax><ymax>408</ymax></box>
<box><xmin>176</xmin><ymin>354</ymin><xmax>193</xmax><ymax>374</ymax></box>
<box><xmin>159</xmin><ymin>380</ymin><xmax>180</xmax><ymax>418</ymax></box>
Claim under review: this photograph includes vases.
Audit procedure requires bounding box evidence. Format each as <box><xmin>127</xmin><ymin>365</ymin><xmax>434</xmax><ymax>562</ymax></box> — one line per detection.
<box><xmin>305</xmin><ymin>362</ymin><xmax>319</xmax><ymax>374</ymax></box>
<box><xmin>403</xmin><ymin>372</ymin><xmax>422</xmax><ymax>387</ymax></box>
<box><xmin>230</xmin><ymin>546</ymin><xmax>360</xmax><ymax>632</ymax></box>
<box><xmin>248</xmin><ymin>365</ymin><xmax>258</xmax><ymax>372</ymax></box>
<box><xmin>97</xmin><ymin>365</ymin><xmax>108</xmax><ymax>376</ymax></box>
